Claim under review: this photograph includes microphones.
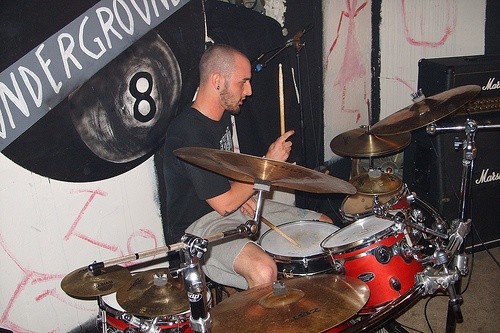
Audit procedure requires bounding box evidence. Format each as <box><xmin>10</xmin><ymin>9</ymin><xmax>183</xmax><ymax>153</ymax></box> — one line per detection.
<box><xmin>251</xmin><ymin>54</ymin><xmax>265</xmax><ymax>70</ymax></box>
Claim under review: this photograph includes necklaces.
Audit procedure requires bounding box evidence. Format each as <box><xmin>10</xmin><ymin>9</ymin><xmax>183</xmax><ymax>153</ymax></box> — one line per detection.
<box><xmin>194</xmin><ymin>101</ymin><xmax>207</xmax><ymax>116</ymax></box>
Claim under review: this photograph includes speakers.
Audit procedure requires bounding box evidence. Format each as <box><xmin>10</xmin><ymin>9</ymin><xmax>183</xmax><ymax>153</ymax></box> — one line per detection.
<box><xmin>402</xmin><ymin>114</ymin><xmax>500</xmax><ymax>254</ymax></box>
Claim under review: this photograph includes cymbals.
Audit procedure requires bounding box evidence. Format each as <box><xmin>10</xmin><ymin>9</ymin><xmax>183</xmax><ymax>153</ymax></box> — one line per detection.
<box><xmin>369</xmin><ymin>84</ymin><xmax>483</xmax><ymax>136</ymax></box>
<box><xmin>171</xmin><ymin>146</ymin><xmax>357</xmax><ymax>196</ymax></box>
<box><xmin>209</xmin><ymin>274</ymin><xmax>371</xmax><ymax>332</ymax></box>
<box><xmin>60</xmin><ymin>263</ymin><xmax>132</xmax><ymax>298</ymax></box>
<box><xmin>329</xmin><ymin>124</ymin><xmax>413</xmax><ymax>159</ymax></box>
<box><xmin>116</xmin><ymin>268</ymin><xmax>192</xmax><ymax>317</ymax></box>
<box><xmin>348</xmin><ymin>171</ymin><xmax>402</xmax><ymax>196</ymax></box>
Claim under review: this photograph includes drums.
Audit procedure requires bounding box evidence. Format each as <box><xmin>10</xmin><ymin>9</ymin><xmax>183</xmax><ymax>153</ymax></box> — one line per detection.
<box><xmin>257</xmin><ymin>217</ymin><xmax>346</xmax><ymax>279</ymax></box>
<box><xmin>318</xmin><ymin>213</ymin><xmax>429</xmax><ymax>316</ymax></box>
<box><xmin>340</xmin><ymin>182</ymin><xmax>423</xmax><ymax>247</ymax></box>
<box><xmin>97</xmin><ymin>283</ymin><xmax>213</xmax><ymax>333</ymax></box>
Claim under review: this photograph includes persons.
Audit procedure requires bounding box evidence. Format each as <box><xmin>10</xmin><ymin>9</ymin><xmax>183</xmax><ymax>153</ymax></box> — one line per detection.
<box><xmin>154</xmin><ymin>43</ymin><xmax>334</xmax><ymax>292</ymax></box>
